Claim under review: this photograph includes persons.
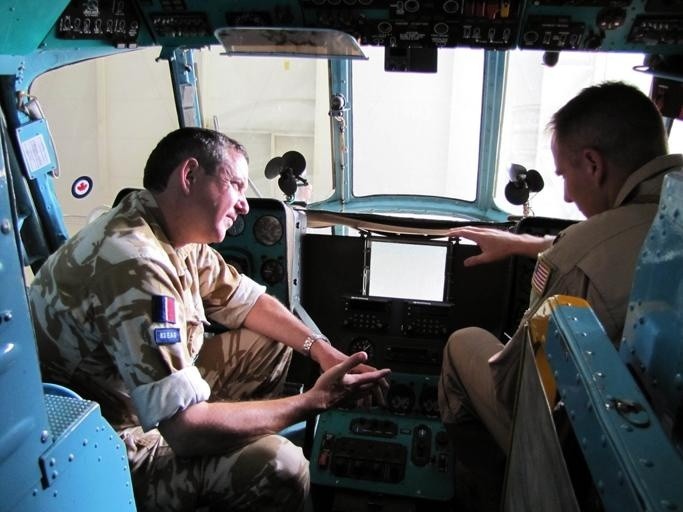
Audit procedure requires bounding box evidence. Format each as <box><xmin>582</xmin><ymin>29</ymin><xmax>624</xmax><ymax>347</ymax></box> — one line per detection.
<box><xmin>28</xmin><ymin>127</ymin><xmax>391</xmax><ymax>512</ymax></box>
<box><xmin>437</xmin><ymin>81</ymin><xmax>683</xmax><ymax>456</ymax></box>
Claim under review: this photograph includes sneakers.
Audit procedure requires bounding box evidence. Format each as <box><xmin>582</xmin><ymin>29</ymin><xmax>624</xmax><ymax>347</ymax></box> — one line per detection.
<box><xmin>420</xmin><ymin>384</ymin><xmax>442</xmax><ymax>419</ymax></box>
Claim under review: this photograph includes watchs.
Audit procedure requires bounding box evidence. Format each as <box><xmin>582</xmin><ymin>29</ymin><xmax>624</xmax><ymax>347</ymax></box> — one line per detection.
<box><xmin>302</xmin><ymin>333</ymin><xmax>331</xmax><ymax>366</ymax></box>
